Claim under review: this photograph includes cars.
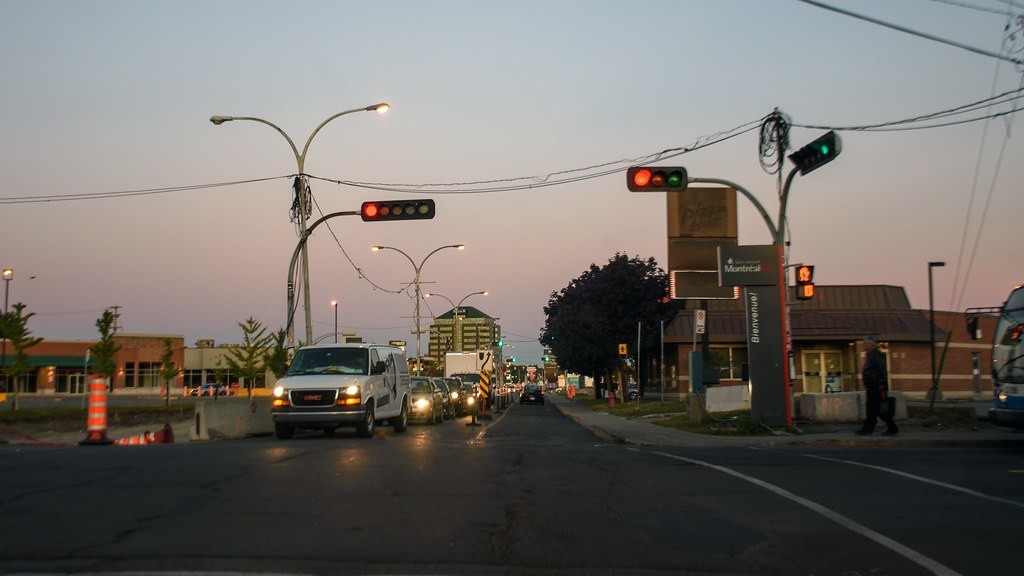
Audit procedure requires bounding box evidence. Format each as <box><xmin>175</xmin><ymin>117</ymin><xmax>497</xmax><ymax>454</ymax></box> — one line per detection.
<box><xmin>408</xmin><ymin>377</ymin><xmax>479</xmax><ymax>426</ymax></box>
<box><xmin>493</xmin><ymin>383</ymin><xmax>523</xmax><ymax>397</ymax></box>
<box><xmin>192</xmin><ymin>383</ymin><xmax>227</xmax><ymax>396</ymax></box>
<box><xmin>520</xmin><ymin>384</ymin><xmax>545</xmax><ymax>405</ymax></box>
<box><xmin>599</xmin><ymin>382</ymin><xmax>642</xmax><ymax>400</ymax></box>
<box><xmin>556</xmin><ymin>386</ymin><xmax>566</xmax><ymax>394</ymax></box>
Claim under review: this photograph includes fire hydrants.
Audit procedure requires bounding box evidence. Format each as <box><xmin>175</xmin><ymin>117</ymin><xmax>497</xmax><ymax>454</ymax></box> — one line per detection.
<box><xmin>608</xmin><ymin>391</ymin><xmax>615</xmax><ymax>408</ymax></box>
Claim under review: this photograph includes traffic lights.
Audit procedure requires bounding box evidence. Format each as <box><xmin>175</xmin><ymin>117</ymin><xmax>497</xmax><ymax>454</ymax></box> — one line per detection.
<box><xmin>361</xmin><ymin>198</ymin><xmax>436</xmax><ymax>223</ymax></box>
<box><xmin>507</xmin><ymin>358</ymin><xmax>516</xmax><ymax>362</ymax></box>
<box><xmin>788</xmin><ymin>132</ymin><xmax>842</xmax><ymax>178</ymax></box>
<box><xmin>492</xmin><ymin>342</ymin><xmax>501</xmax><ymax>348</ymax></box>
<box><xmin>796</xmin><ymin>265</ymin><xmax>815</xmax><ymax>299</ymax></box>
<box><xmin>541</xmin><ymin>358</ymin><xmax>550</xmax><ymax>360</ymax></box>
<box><xmin>627</xmin><ymin>166</ymin><xmax>689</xmax><ymax>193</ymax></box>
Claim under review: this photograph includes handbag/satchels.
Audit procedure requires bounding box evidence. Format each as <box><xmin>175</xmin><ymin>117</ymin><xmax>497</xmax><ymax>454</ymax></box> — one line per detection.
<box><xmin>887</xmin><ymin>396</ymin><xmax>895</xmax><ymax>417</ymax></box>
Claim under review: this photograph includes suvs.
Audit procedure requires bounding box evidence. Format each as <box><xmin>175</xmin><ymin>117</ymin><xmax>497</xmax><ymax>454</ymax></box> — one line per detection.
<box><xmin>271</xmin><ymin>343</ymin><xmax>413</xmax><ymax>437</ymax></box>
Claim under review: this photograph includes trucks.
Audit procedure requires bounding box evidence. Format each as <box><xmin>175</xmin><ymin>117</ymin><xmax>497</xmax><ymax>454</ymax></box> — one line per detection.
<box><xmin>445</xmin><ymin>350</ymin><xmax>494</xmax><ymax>406</ymax></box>
<box><xmin>965</xmin><ymin>283</ymin><xmax>1024</xmax><ymax>433</ymax></box>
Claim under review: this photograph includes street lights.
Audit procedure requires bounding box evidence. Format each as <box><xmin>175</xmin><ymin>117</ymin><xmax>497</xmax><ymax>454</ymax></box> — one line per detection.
<box><xmin>331</xmin><ymin>302</ymin><xmax>338</xmax><ymax>344</ymax></box>
<box><xmin>424</xmin><ymin>291</ymin><xmax>489</xmax><ymax>353</ymax></box>
<box><xmin>210</xmin><ymin>103</ymin><xmax>391</xmax><ymax>345</ymax></box>
<box><xmin>2</xmin><ymin>268</ymin><xmax>15</xmax><ymax>313</ymax></box>
<box><xmin>564</xmin><ymin>370</ymin><xmax>568</xmax><ymax>392</ymax></box>
<box><xmin>371</xmin><ymin>244</ymin><xmax>465</xmax><ymax>377</ymax></box>
<box><xmin>928</xmin><ymin>261</ymin><xmax>946</xmax><ymax>400</ymax></box>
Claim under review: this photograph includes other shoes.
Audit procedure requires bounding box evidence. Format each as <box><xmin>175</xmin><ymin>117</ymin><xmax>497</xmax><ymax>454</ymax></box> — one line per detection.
<box><xmin>854</xmin><ymin>430</ymin><xmax>873</xmax><ymax>436</ymax></box>
<box><xmin>879</xmin><ymin>429</ymin><xmax>898</xmax><ymax>436</ymax></box>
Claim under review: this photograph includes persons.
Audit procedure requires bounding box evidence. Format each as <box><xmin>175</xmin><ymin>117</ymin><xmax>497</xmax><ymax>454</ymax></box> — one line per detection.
<box><xmin>854</xmin><ymin>336</ymin><xmax>900</xmax><ymax>436</ymax></box>
